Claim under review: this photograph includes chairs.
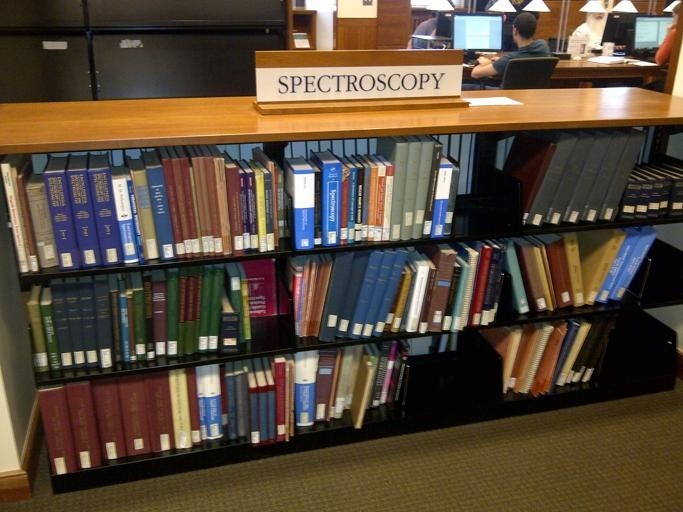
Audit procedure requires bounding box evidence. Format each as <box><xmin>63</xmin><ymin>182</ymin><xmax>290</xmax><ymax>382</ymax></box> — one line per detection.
<box><xmin>480</xmin><ymin>57</ymin><xmax>559</xmax><ymax>89</ymax></box>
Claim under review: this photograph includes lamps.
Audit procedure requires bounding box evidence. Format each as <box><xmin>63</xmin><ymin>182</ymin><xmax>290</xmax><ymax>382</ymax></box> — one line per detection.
<box><xmin>521</xmin><ymin>0</ymin><xmax>551</xmax><ymax>13</ymax></box>
<box><xmin>578</xmin><ymin>0</ymin><xmax>608</xmax><ymax>13</ymax></box>
<box><xmin>663</xmin><ymin>0</ymin><xmax>681</xmax><ymax>13</ymax></box>
<box><xmin>425</xmin><ymin>0</ymin><xmax>455</xmax><ymax>12</ymax></box>
<box><xmin>486</xmin><ymin>0</ymin><xmax>517</xmax><ymax>13</ymax></box>
<box><xmin>611</xmin><ymin>0</ymin><xmax>638</xmax><ymax>13</ymax></box>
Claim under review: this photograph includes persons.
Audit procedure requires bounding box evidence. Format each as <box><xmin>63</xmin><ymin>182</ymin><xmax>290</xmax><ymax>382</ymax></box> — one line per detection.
<box><xmin>568</xmin><ymin>17</ymin><xmax>607</xmax><ymax>55</ymax></box>
<box><xmin>655</xmin><ymin>2</ymin><xmax>683</xmax><ymax>65</ymax></box>
<box><xmin>407</xmin><ymin>13</ymin><xmax>453</xmax><ymax>50</ymax></box>
<box><xmin>470</xmin><ymin>13</ymin><xmax>551</xmax><ymax>79</ymax></box>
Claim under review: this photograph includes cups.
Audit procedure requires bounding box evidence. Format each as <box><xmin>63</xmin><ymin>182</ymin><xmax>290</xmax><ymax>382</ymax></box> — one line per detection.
<box><xmin>602</xmin><ymin>42</ymin><xmax>614</xmax><ymax>55</ymax></box>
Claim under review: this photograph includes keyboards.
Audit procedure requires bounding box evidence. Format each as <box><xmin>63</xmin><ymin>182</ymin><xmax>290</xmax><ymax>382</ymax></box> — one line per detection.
<box><xmin>591</xmin><ymin>49</ymin><xmax>625</xmax><ymax>53</ymax></box>
<box><xmin>464</xmin><ymin>55</ymin><xmax>479</xmax><ymax>65</ymax></box>
<box><xmin>640</xmin><ymin>56</ymin><xmax>655</xmax><ymax>62</ymax></box>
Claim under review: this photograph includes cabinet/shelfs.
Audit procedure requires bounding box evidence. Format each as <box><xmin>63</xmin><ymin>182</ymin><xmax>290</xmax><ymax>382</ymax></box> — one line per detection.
<box><xmin>0</xmin><ymin>87</ymin><xmax>683</xmax><ymax>496</ymax></box>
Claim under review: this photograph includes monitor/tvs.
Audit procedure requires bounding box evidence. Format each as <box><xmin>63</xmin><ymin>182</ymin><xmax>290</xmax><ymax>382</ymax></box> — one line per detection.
<box><xmin>600</xmin><ymin>11</ymin><xmax>652</xmax><ymax>56</ymax></box>
<box><xmin>452</xmin><ymin>13</ymin><xmax>503</xmax><ymax>55</ymax></box>
<box><xmin>632</xmin><ymin>15</ymin><xmax>676</xmax><ymax>51</ymax></box>
<box><xmin>436</xmin><ymin>12</ymin><xmax>464</xmax><ymax>37</ymax></box>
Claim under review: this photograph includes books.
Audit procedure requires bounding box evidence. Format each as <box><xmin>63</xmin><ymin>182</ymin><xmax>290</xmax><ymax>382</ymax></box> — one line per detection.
<box><xmin>1</xmin><ymin>133</ymin><xmax>459</xmax><ymax>475</ymax></box>
<box><xmin>451</xmin><ymin>135</ymin><xmax>683</xmax><ymax>398</ymax></box>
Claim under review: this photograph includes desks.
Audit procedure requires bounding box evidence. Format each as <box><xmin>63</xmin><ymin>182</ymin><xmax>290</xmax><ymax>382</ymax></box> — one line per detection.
<box><xmin>462</xmin><ymin>53</ymin><xmax>669</xmax><ymax>87</ymax></box>
